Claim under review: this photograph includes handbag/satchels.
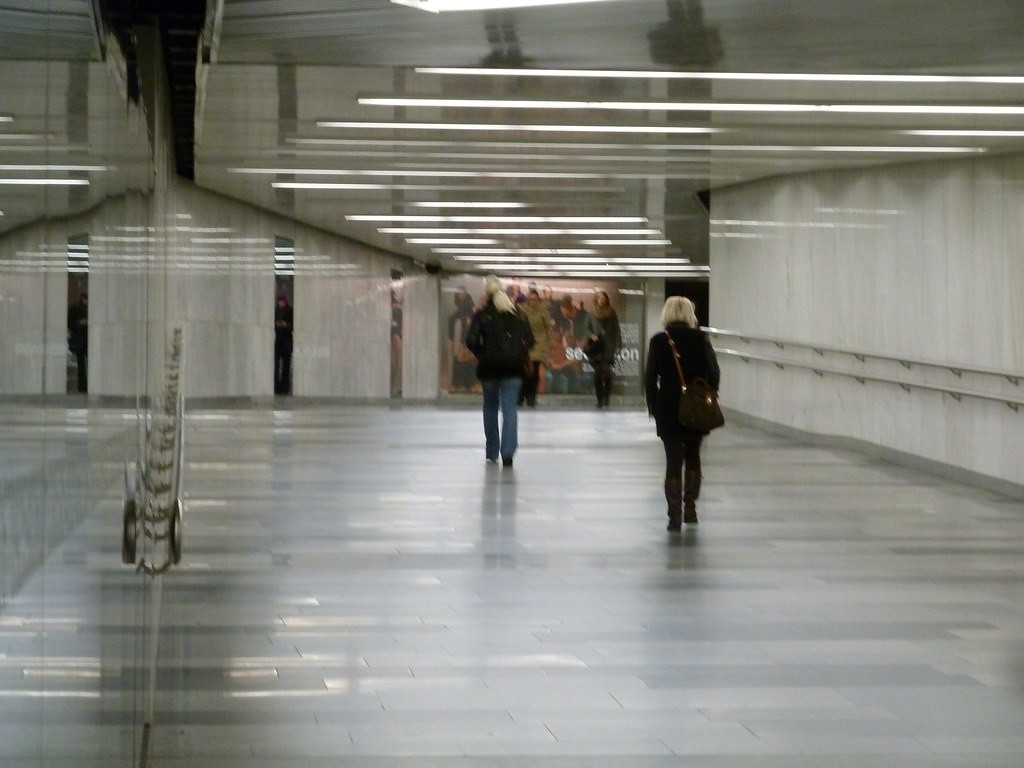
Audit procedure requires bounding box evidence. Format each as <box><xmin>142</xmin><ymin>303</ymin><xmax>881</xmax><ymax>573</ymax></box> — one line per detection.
<box><xmin>679</xmin><ymin>386</ymin><xmax>725</xmax><ymax>428</ymax></box>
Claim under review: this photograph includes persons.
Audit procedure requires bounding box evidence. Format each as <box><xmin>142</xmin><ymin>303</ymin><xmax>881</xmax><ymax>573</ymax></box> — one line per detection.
<box><xmin>274</xmin><ymin>298</ymin><xmax>293</xmax><ymax>397</ymax></box>
<box><xmin>466</xmin><ymin>279</ymin><xmax>535</xmax><ymax>467</ymax></box>
<box><xmin>517</xmin><ymin>290</ymin><xmax>555</xmax><ymax>408</ymax></box>
<box><xmin>584</xmin><ymin>292</ymin><xmax>623</xmax><ymax>407</ymax></box>
<box><xmin>645</xmin><ymin>297</ymin><xmax>721</xmax><ymax>531</ymax></box>
<box><xmin>67</xmin><ymin>294</ymin><xmax>87</xmax><ymax>393</ymax></box>
<box><xmin>447</xmin><ymin>283</ymin><xmax>596</xmax><ymax>396</ymax></box>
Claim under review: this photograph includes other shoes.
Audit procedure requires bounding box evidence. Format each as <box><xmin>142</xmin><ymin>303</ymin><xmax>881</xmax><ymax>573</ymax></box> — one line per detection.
<box><xmin>684</xmin><ymin>510</ymin><xmax>698</xmax><ymax>523</ymax></box>
<box><xmin>667</xmin><ymin>520</ymin><xmax>681</xmax><ymax>531</ymax></box>
<box><xmin>486</xmin><ymin>453</ymin><xmax>497</xmax><ymax>462</ymax></box>
<box><xmin>503</xmin><ymin>455</ymin><xmax>513</xmax><ymax>467</ymax></box>
